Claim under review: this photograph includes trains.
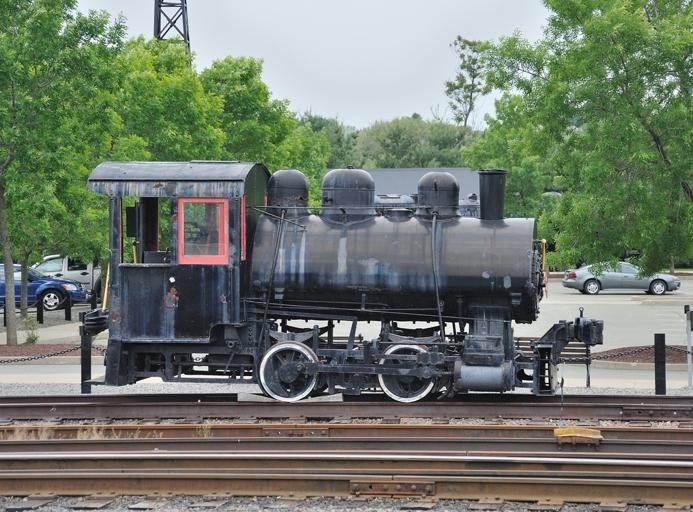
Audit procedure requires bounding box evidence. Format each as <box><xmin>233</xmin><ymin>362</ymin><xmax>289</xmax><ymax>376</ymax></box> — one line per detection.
<box><xmin>79</xmin><ymin>160</ymin><xmax>603</xmax><ymax>402</ymax></box>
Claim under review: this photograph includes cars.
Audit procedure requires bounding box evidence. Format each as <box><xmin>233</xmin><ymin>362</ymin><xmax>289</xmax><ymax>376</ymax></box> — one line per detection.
<box><xmin>562</xmin><ymin>261</ymin><xmax>681</xmax><ymax>295</ymax></box>
<box><xmin>0</xmin><ymin>253</ymin><xmax>101</xmax><ymax>311</ymax></box>
